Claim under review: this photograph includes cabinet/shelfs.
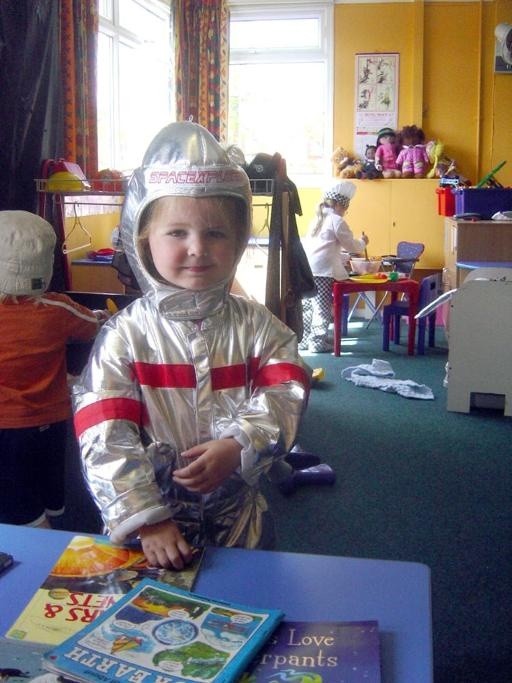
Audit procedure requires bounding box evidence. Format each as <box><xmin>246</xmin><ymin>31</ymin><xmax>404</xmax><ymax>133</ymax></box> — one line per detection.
<box><xmin>335</xmin><ymin>176</ymin><xmax>463</xmax><ymax>310</ymax></box>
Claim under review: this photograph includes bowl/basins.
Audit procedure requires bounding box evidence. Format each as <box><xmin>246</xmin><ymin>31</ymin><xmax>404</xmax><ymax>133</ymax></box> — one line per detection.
<box><xmin>348</xmin><ymin>257</ymin><xmax>383</xmax><ymax>274</ymax></box>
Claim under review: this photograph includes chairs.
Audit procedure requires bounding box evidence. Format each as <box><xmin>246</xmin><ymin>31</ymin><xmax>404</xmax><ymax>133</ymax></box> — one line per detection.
<box><xmin>347</xmin><ymin>241</ymin><xmax>425</xmax><ymax>329</ymax></box>
<box><xmin>382</xmin><ymin>272</ymin><xmax>440</xmax><ymax>356</ymax></box>
<box><xmin>331</xmin><ymin>294</ymin><xmax>349</xmax><ymax>336</ymax></box>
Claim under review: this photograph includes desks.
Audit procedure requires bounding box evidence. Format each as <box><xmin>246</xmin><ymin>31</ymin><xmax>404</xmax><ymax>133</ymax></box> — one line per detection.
<box><xmin>455</xmin><ymin>261</ymin><xmax>512</xmax><ymax>271</ymax></box>
<box><xmin>332</xmin><ymin>274</ymin><xmax>421</xmax><ymax>356</ymax></box>
<box><xmin>0</xmin><ymin>521</ymin><xmax>436</xmax><ymax>682</ymax></box>
<box><xmin>443</xmin><ymin>217</ymin><xmax>512</xmax><ymax>289</ymax></box>
<box><xmin>71</xmin><ymin>259</ymin><xmax>126</xmax><ymax>295</ymax></box>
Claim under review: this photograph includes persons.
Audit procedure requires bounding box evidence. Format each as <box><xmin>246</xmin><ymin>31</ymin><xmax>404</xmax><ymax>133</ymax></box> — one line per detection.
<box><xmin>0</xmin><ymin>209</ymin><xmax>109</xmax><ymax>533</ymax></box>
<box><xmin>70</xmin><ymin>121</ymin><xmax>315</xmax><ymax>571</ymax></box>
<box><xmin>296</xmin><ymin>178</ymin><xmax>370</xmax><ymax>353</ymax></box>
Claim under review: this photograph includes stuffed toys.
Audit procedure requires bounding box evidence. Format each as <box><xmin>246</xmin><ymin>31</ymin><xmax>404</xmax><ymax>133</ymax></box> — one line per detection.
<box><xmin>327</xmin><ymin>122</ymin><xmax>455</xmax><ymax>177</ymax></box>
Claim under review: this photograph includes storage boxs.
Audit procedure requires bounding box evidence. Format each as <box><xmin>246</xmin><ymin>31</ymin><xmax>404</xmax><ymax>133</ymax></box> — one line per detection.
<box><xmin>452</xmin><ymin>188</ymin><xmax>512</xmax><ymax>219</ymax></box>
<box><xmin>435</xmin><ymin>187</ymin><xmax>454</xmax><ymax>217</ymax></box>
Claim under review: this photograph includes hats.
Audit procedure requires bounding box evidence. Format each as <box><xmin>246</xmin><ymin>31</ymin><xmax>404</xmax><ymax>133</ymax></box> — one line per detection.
<box><xmin>0</xmin><ymin>210</ymin><xmax>57</xmax><ymax>297</ymax></box>
<box><xmin>323</xmin><ymin>180</ymin><xmax>357</xmax><ymax>210</ymax></box>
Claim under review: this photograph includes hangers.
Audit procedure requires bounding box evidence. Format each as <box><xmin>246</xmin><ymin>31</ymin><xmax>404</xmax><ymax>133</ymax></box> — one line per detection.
<box><xmin>61</xmin><ymin>201</ymin><xmax>92</xmax><ymax>254</ymax></box>
<box><xmin>254</xmin><ymin>206</ymin><xmax>273</xmax><ymax>256</ymax></box>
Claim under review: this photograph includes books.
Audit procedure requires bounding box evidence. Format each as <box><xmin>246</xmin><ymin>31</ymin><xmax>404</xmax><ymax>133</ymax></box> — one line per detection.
<box><xmin>0</xmin><ymin>535</ymin><xmax>383</xmax><ymax>682</ymax></box>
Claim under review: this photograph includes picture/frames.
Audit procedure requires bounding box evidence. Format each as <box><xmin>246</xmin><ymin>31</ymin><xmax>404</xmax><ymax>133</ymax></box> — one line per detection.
<box><xmin>353</xmin><ymin>53</ymin><xmax>400</xmax><ymax>164</ymax></box>
